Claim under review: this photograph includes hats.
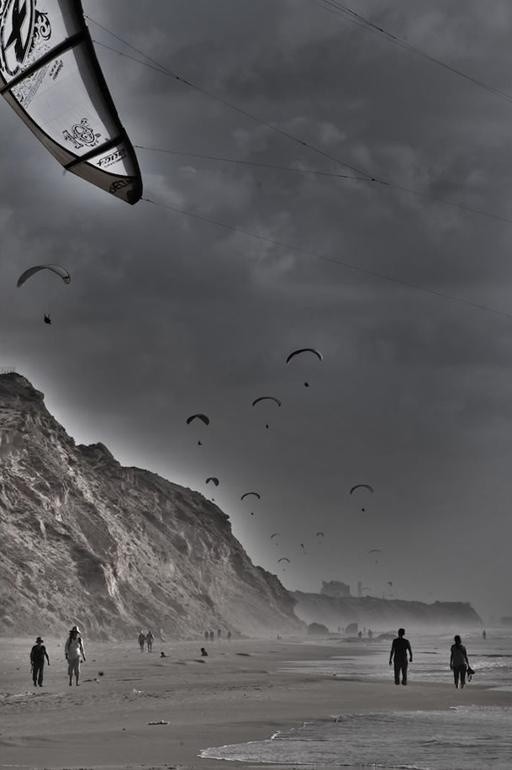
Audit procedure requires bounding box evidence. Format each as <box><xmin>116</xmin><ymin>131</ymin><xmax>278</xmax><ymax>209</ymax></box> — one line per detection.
<box><xmin>35</xmin><ymin>637</ymin><xmax>43</xmax><ymax>642</ymax></box>
<box><xmin>69</xmin><ymin>626</ymin><xmax>80</xmax><ymax>633</ymax></box>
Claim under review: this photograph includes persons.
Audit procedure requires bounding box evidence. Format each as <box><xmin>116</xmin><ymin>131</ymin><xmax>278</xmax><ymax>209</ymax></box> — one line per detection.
<box><xmin>30</xmin><ymin>637</ymin><xmax>51</xmax><ymax>689</ymax></box>
<box><xmin>144</xmin><ymin>631</ymin><xmax>155</xmax><ymax>652</ymax></box>
<box><xmin>203</xmin><ymin>628</ymin><xmax>233</xmax><ymax>643</ymax></box>
<box><xmin>200</xmin><ymin>647</ymin><xmax>208</xmax><ymax>656</ymax></box>
<box><xmin>449</xmin><ymin>634</ymin><xmax>471</xmax><ymax>689</ymax></box>
<box><xmin>137</xmin><ymin>630</ymin><xmax>147</xmax><ymax>651</ymax></box>
<box><xmin>63</xmin><ymin>625</ymin><xmax>87</xmax><ymax>688</ymax></box>
<box><xmin>387</xmin><ymin>626</ymin><xmax>414</xmax><ymax>686</ymax></box>
